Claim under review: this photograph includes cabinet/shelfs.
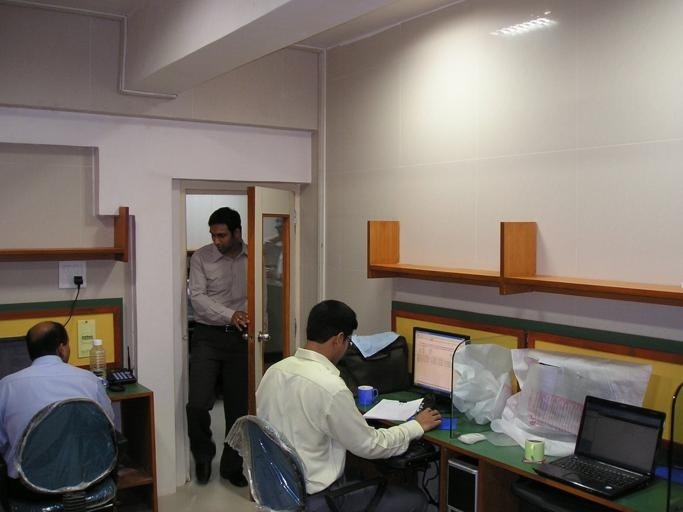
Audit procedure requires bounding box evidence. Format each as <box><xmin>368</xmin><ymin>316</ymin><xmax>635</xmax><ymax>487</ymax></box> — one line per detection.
<box><xmin>89</xmin><ymin>377</ymin><xmax>159</xmax><ymax>512</ymax></box>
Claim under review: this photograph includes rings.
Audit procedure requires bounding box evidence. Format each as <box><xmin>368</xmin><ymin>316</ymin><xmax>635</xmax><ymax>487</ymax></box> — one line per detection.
<box><xmin>236</xmin><ymin>319</ymin><xmax>239</xmax><ymax>321</ymax></box>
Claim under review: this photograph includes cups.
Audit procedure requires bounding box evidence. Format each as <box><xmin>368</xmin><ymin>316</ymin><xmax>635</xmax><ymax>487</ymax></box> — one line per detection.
<box><xmin>525</xmin><ymin>440</ymin><xmax>544</xmax><ymax>463</ymax></box>
<box><xmin>358</xmin><ymin>385</ymin><xmax>378</xmax><ymax>405</ymax></box>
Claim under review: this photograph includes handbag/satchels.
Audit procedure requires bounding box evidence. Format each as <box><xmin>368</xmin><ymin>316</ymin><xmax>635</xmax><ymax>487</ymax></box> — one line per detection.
<box><xmin>334</xmin><ymin>333</ymin><xmax>410</xmax><ymax>399</ymax></box>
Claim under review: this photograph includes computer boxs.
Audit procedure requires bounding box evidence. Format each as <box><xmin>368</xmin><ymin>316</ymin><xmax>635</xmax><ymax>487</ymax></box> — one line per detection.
<box><xmin>448</xmin><ymin>458</ymin><xmax>479</xmax><ymax>512</ymax></box>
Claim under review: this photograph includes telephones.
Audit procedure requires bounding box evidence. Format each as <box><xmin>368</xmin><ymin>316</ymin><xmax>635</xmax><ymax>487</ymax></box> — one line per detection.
<box><xmin>105</xmin><ymin>366</ymin><xmax>137</xmax><ymax>387</ymax></box>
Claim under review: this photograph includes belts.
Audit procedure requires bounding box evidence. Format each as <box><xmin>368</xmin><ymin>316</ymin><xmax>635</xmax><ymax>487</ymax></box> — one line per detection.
<box><xmin>192</xmin><ymin>319</ymin><xmax>248</xmax><ymax>334</ymax></box>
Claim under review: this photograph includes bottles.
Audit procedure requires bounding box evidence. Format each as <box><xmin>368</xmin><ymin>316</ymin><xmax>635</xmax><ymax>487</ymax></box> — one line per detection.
<box><xmin>88</xmin><ymin>339</ymin><xmax>107</xmax><ymax>380</ymax></box>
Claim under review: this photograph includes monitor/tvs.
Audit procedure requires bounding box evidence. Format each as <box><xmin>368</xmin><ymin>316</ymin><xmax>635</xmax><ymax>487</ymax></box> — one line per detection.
<box><xmin>409</xmin><ymin>326</ymin><xmax>471</xmax><ymax>412</ymax></box>
<box><xmin>0</xmin><ymin>335</ymin><xmax>33</xmax><ymax>382</ymax></box>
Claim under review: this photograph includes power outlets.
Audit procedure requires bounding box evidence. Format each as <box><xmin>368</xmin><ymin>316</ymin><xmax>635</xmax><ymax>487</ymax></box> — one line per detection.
<box><xmin>58</xmin><ymin>260</ymin><xmax>87</xmax><ymax>289</ymax></box>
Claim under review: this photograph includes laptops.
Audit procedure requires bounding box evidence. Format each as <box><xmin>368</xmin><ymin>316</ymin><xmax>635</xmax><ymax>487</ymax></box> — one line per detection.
<box><xmin>533</xmin><ymin>395</ymin><xmax>666</xmax><ymax>500</ymax></box>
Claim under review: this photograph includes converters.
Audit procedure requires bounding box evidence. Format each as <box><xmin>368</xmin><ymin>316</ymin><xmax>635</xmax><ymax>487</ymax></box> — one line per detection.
<box><xmin>75</xmin><ymin>276</ymin><xmax>83</xmax><ymax>285</ymax></box>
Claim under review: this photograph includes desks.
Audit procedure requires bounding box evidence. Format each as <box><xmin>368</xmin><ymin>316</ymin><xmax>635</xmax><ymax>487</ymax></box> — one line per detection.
<box><xmin>353</xmin><ymin>386</ymin><xmax>682</xmax><ymax>512</ymax></box>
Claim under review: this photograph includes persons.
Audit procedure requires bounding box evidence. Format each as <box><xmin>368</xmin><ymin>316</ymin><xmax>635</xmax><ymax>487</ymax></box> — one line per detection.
<box><xmin>257</xmin><ymin>300</ymin><xmax>442</xmax><ymax>512</ymax></box>
<box><xmin>186</xmin><ymin>208</ymin><xmax>249</xmax><ymax>487</ymax></box>
<box><xmin>0</xmin><ymin>321</ymin><xmax>119</xmax><ymax>505</ymax></box>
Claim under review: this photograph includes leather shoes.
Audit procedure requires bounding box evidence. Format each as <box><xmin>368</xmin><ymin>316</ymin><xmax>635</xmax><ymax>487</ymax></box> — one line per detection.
<box><xmin>218</xmin><ymin>464</ymin><xmax>248</xmax><ymax>488</ymax></box>
<box><xmin>194</xmin><ymin>440</ymin><xmax>216</xmax><ymax>485</ymax></box>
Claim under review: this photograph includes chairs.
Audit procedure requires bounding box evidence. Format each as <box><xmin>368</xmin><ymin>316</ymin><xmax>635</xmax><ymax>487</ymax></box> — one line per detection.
<box><xmin>241</xmin><ymin>414</ymin><xmax>388</xmax><ymax>512</ymax></box>
<box><xmin>0</xmin><ymin>398</ymin><xmax>121</xmax><ymax>512</ymax></box>
